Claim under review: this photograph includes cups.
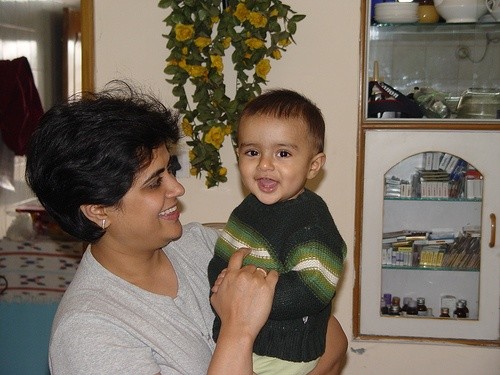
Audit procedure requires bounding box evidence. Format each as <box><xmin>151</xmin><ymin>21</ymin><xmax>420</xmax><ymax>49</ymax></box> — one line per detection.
<box><xmin>485</xmin><ymin>0</ymin><xmax>500</xmax><ymax>22</ymax></box>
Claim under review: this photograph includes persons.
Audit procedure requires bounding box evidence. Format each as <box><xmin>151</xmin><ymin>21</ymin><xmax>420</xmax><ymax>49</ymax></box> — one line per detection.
<box><xmin>24</xmin><ymin>82</ymin><xmax>348</xmax><ymax>374</ymax></box>
<box><xmin>208</xmin><ymin>90</ymin><xmax>349</xmax><ymax>375</ymax></box>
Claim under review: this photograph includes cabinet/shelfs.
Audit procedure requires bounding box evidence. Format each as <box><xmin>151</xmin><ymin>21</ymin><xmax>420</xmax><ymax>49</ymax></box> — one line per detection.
<box><xmin>352</xmin><ymin>0</ymin><xmax>500</xmax><ymax>349</ymax></box>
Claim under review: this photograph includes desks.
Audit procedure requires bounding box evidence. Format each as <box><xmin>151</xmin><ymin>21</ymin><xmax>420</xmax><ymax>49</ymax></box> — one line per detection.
<box><xmin>15</xmin><ymin>205</ymin><xmax>64</xmax><ymax>242</ymax></box>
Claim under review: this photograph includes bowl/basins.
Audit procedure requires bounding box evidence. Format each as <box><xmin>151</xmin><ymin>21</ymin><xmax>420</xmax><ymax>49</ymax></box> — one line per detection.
<box><xmin>433</xmin><ymin>0</ymin><xmax>490</xmax><ymax>24</ymax></box>
<box><xmin>374</xmin><ymin>2</ymin><xmax>419</xmax><ymax>24</ymax></box>
<box><xmin>417</xmin><ymin>0</ymin><xmax>438</xmax><ymax>24</ymax></box>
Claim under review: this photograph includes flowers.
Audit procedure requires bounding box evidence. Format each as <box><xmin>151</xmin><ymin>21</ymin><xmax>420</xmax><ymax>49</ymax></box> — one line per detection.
<box><xmin>155</xmin><ymin>0</ymin><xmax>306</xmax><ymax>194</ymax></box>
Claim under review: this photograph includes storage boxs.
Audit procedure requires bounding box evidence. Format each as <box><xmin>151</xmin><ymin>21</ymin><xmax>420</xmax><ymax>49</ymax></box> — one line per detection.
<box><xmin>464</xmin><ymin>169</ymin><xmax>484</xmax><ymax>200</ymax></box>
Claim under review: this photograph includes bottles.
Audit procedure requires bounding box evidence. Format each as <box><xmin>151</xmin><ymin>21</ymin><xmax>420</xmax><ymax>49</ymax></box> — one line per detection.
<box><xmin>380</xmin><ymin>293</ymin><xmax>470</xmax><ymax>319</ymax></box>
